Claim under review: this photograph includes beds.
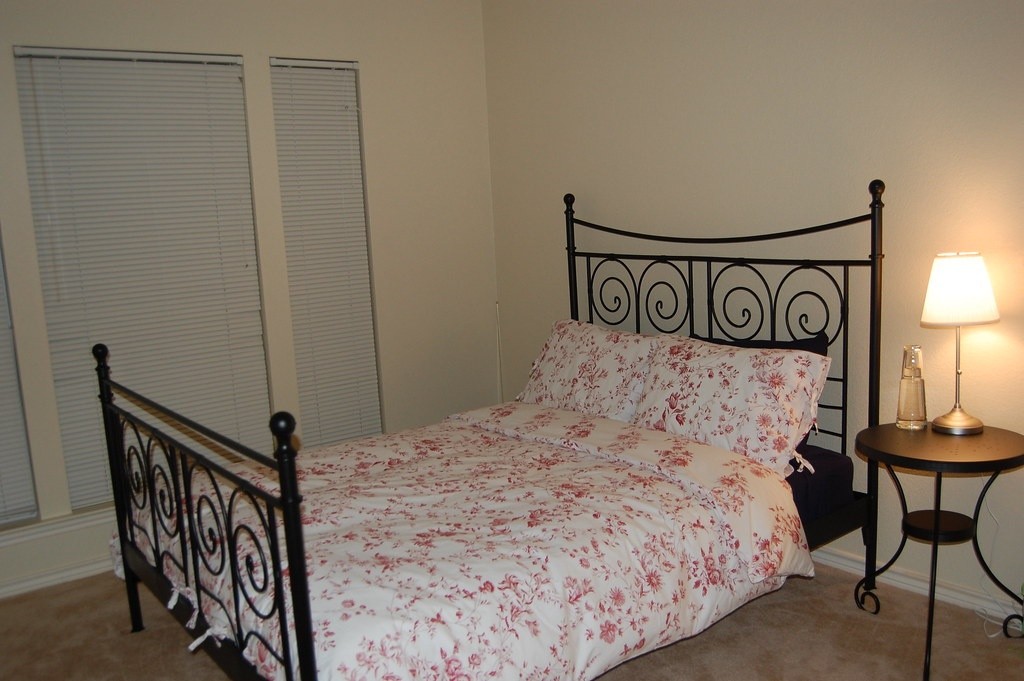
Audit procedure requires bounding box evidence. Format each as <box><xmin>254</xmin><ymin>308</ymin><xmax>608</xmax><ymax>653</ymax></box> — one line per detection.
<box><xmin>91</xmin><ymin>177</ymin><xmax>885</xmax><ymax>681</ymax></box>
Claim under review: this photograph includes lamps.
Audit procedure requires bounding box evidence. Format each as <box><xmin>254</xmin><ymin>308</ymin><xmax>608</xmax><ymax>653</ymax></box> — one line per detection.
<box><xmin>919</xmin><ymin>251</ymin><xmax>1001</xmax><ymax>436</ymax></box>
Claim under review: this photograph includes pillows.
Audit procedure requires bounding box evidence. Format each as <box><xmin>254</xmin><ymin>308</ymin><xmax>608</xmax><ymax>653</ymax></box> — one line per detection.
<box><xmin>631</xmin><ymin>333</ymin><xmax>833</xmax><ymax>480</ymax></box>
<box><xmin>688</xmin><ymin>334</ymin><xmax>829</xmax><ymax>465</ymax></box>
<box><xmin>516</xmin><ymin>319</ymin><xmax>658</xmax><ymax>426</ymax></box>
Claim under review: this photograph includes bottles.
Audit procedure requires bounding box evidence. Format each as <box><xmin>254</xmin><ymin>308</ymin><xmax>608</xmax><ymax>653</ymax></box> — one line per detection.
<box><xmin>896</xmin><ymin>345</ymin><xmax>928</xmax><ymax>431</ymax></box>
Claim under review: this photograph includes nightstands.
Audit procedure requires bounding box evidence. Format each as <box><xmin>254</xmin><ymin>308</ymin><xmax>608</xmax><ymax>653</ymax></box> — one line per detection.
<box><xmin>853</xmin><ymin>423</ymin><xmax>1024</xmax><ymax>681</ymax></box>
